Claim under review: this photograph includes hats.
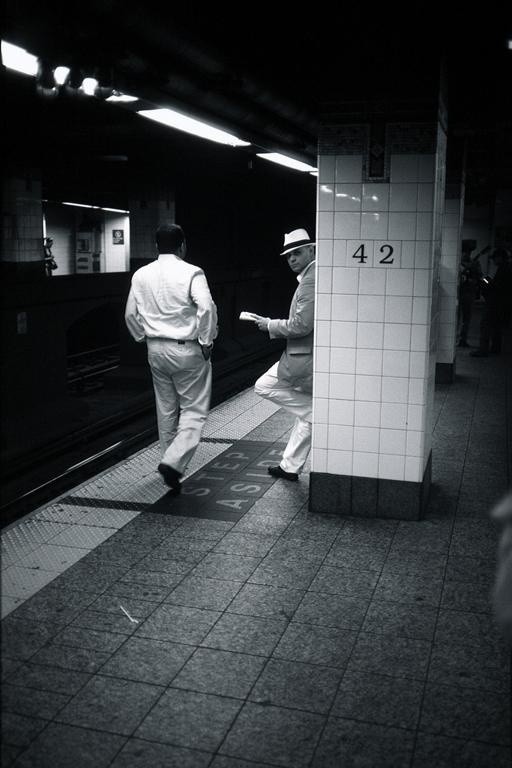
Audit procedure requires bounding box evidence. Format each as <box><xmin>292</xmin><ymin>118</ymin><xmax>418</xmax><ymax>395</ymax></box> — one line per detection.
<box><xmin>279</xmin><ymin>229</ymin><xmax>316</xmax><ymax>255</ymax></box>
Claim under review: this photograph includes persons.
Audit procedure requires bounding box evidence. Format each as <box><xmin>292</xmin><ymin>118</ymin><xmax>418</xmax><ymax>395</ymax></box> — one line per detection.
<box><xmin>124</xmin><ymin>224</ymin><xmax>218</xmax><ymax>492</ymax></box>
<box><xmin>455</xmin><ymin>245</ymin><xmax>481</xmax><ymax>346</ymax></box>
<box><xmin>43</xmin><ymin>238</ymin><xmax>54</xmax><ymax>276</ymax></box>
<box><xmin>470</xmin><ymin>248</ymin><xmax>512</xmax><ymax>356</ymax></box>
<box><xmin>251</xmin><ymin>228</ymin><xmax>315</xmax><ymax>481</ymax></box>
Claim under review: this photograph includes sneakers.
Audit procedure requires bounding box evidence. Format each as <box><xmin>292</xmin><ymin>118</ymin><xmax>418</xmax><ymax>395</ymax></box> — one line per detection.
<box><xmin>158</xmin><ymin>464</ymin><xmax>181</xmax><ymax>491</ymax></box>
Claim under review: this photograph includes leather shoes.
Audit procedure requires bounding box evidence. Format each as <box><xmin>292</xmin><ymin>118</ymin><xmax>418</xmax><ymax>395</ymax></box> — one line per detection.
<box><xmin>268</xmin><ymin>465</ymin><xmax>298</xmax><ymax>481</ymax></box>
